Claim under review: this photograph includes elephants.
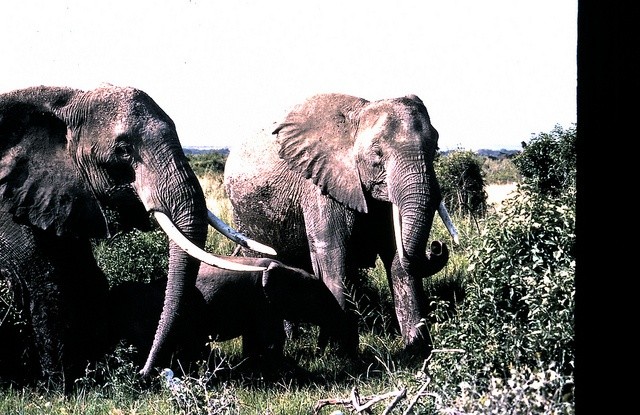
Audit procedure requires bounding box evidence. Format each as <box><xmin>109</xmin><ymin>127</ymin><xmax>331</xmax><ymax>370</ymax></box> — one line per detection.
<box><xmin>224</xmin><ymin>94</ymin><xmax>460</xmax><ymax>353</ymax></box>
<box><xmin>195</xmin><ymin>253</ymin><xmax>359</xmax><ymax>361</ymax></box>
<box><xmin>0</xmin><ymin>85</ymin><xmax>277</xmax><ymax>396</ymax></box>
